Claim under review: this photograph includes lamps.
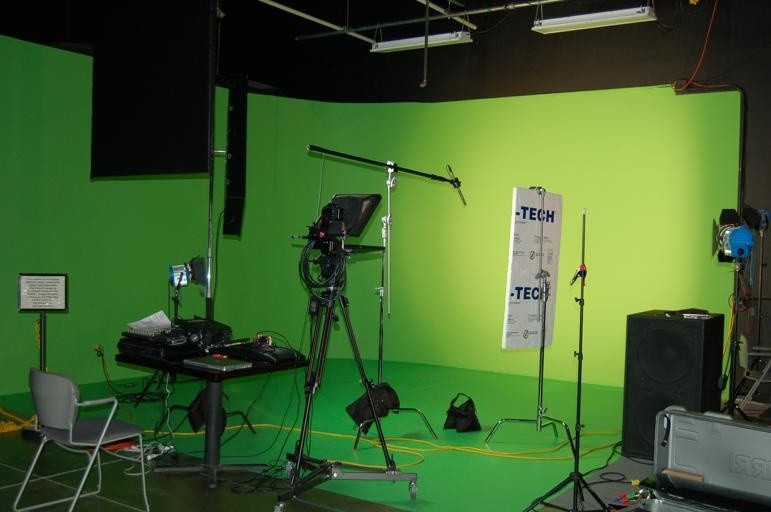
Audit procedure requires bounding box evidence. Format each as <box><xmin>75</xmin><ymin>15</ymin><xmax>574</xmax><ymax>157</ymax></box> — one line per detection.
<box><xmin>369</xmin><ymin>11</ymin><xmax>475</xmax><ymax>54</ymax></box>
<box><xmin>530</xmin><ymin>0</ymin><xmax>660</xmax><ymax>37</ymax></box>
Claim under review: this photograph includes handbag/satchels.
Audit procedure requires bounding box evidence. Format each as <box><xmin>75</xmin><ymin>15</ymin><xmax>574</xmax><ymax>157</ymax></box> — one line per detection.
<box><xmin>442</xmin><ymin>391</ymin><xmax>482</xmax><ymax>434</ymax></box>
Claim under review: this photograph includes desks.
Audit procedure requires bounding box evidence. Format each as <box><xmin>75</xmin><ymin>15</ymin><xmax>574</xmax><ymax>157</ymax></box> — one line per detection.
<box><xmin>116</xmin><ymin>339</ymin><xmax>310</xmax><ymax>480</ymax></box>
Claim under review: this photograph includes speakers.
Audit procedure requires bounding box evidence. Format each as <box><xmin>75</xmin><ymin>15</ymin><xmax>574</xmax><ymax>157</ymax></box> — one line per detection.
<box><xmin>620</xmin><ymin>305</ymin><xmax>725</xmax><ymax>460</ymax></box>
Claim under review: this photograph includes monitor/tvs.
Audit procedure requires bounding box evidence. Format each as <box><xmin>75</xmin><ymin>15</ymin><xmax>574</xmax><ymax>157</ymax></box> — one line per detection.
<box><xmin>311</xmin><ymin>193</ymin><xmax>383</xmax><ymax>236</ymax></box>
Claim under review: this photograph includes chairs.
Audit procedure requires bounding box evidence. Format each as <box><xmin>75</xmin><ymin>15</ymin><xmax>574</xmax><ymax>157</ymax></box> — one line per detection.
<box><xmin>11</xmin><ymin>366</ymin><xmax>152</xmax><ymax>511</ymax></box>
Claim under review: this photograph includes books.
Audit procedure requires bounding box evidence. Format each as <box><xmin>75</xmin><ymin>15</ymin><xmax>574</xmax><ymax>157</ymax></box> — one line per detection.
<box><xmin>127</xmin><ymin>311</ymin><xmax>178</xmax><ymax>339</ymax></box>
<box><xmin>183</xmin><ymin>355</ymin><xmax>255</xmax><ymax>373</ymax></box>
